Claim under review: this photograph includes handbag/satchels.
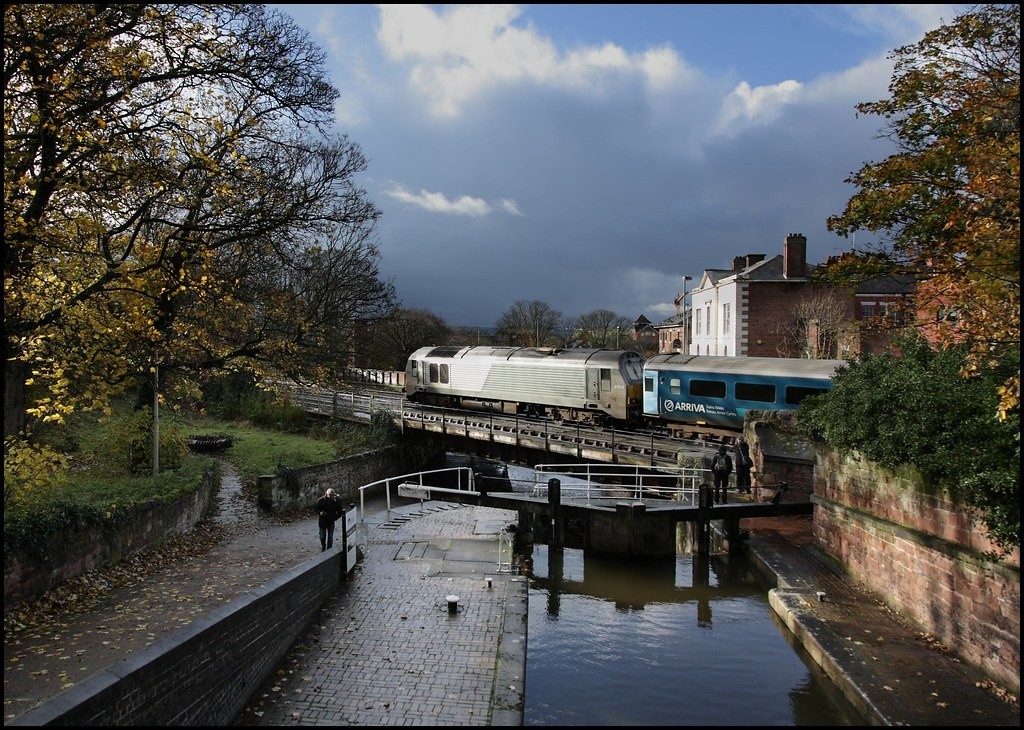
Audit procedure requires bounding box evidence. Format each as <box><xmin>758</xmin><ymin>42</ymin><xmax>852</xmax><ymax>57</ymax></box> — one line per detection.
<box><xmin>743</xmin><ymin>456</ymin><xmax>753</xmax><ymax>468</ymax></box>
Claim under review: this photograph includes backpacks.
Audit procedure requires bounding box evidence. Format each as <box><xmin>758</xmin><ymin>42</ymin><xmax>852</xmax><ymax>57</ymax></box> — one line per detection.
<box><xmin>714</xmin><ymin>454</ymin><xmax>727</xmax><ymax>472</ymax></box>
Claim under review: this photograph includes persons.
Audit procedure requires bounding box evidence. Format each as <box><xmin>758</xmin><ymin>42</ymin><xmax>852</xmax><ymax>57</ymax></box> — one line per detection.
<box><xmin>710</xmin><ymin>445</ymin><xmax>732</xmax><ymax>503</ymax></box>
<box><xmin>732</xmin><ymin>435</ymin><xmax>754</xmax><ymax>494</ymax></box>
<box><xmin>314</xmin><ymin>486</ymin><xmax>343</xmax><ymax>552</ymax></box>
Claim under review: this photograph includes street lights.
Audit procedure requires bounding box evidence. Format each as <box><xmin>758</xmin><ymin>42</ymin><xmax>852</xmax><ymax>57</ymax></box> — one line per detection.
<box><xmin>681</xmin><ymin>274</ymin><xmax>692</xmax><ymax>354</ymax></box>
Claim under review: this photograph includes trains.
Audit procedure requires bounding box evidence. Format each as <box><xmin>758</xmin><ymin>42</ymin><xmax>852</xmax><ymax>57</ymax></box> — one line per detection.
<box><xmin>400</xmin><ymin>343</ymin><xmax>856</xmax><ymax>452</ymax></box>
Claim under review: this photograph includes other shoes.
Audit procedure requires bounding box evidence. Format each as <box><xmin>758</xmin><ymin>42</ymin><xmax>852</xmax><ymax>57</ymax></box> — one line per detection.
<box><xmin>723</xmin><ymin>501</ymin><xmax>728</xmax><ymax>504</ymax></box>
<box><xmin>713</xmin><ymin>501</ymin><xmax>719</xmax><ymax>505</ymax></box>
